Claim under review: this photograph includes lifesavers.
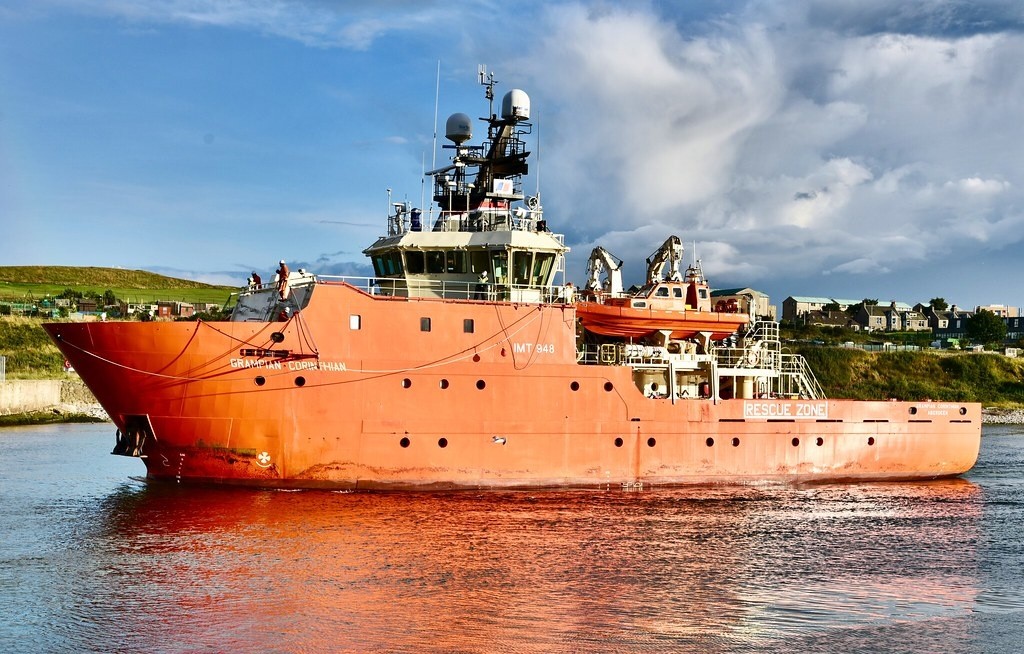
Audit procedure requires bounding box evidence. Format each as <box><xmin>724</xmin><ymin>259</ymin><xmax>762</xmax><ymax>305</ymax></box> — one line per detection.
<box><xmin>747</xmin><ymin>351</ymin><xmax>760</xmax><ymax>366</ymax></box>
<box><xmin>63</xmin><ymin>359</ymin><xmax>71</xmax><ymax>368</ymax></box>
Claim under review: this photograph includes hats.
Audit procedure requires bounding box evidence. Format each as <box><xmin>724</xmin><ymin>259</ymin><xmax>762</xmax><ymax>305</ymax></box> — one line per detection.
<box><xmin>284</xmin><ymin>306</ymin><xmax>290</xmax><ymax>313</ymax></box>
<box><xmin>482</xmin><ymin>271</ymin><xmax>488</xmax><ymax>278</ymax></box>
<box><xmin>251</xmin><ymin>271</ymin><xmax>256</xmax><ymax>274</ymax></box>
<box><xmin>280</xmin><ymin>260</ymin><xmax>285</xmax><ymax>264</ymax></box>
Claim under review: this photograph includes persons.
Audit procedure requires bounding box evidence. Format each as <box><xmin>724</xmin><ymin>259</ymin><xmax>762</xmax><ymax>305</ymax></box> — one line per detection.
<box><xmin>474</xmin><ymin>271</ymin><xmax>488</xmax><ymax>300</ymax></box>
<box><xmin>251</xmin><ymin>271</ymin><xmax>262</xmax><ymax>294</ymax></box>
<box><xmin>278</xmin><ymin>306</ymin><xmax>290</xmax><ymax>322</ymax></box>
<box><xmin>276</xmin><ymin>260</ymin><xmax>288</xmax><ymax>302</ymax></box>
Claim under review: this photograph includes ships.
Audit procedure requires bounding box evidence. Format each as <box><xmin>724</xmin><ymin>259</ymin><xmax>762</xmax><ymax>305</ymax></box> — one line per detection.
<box><xmin>42</xmin><ymin>59</ymin><xmax>982</xmax><ymax>488</ymax></box>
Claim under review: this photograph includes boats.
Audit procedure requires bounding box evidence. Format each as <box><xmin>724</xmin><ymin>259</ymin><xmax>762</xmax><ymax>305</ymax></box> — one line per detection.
<box><xmin>577</xmin><ymin>239</ymin><xmax>749</xmax><ymax>342</ymax></box>
